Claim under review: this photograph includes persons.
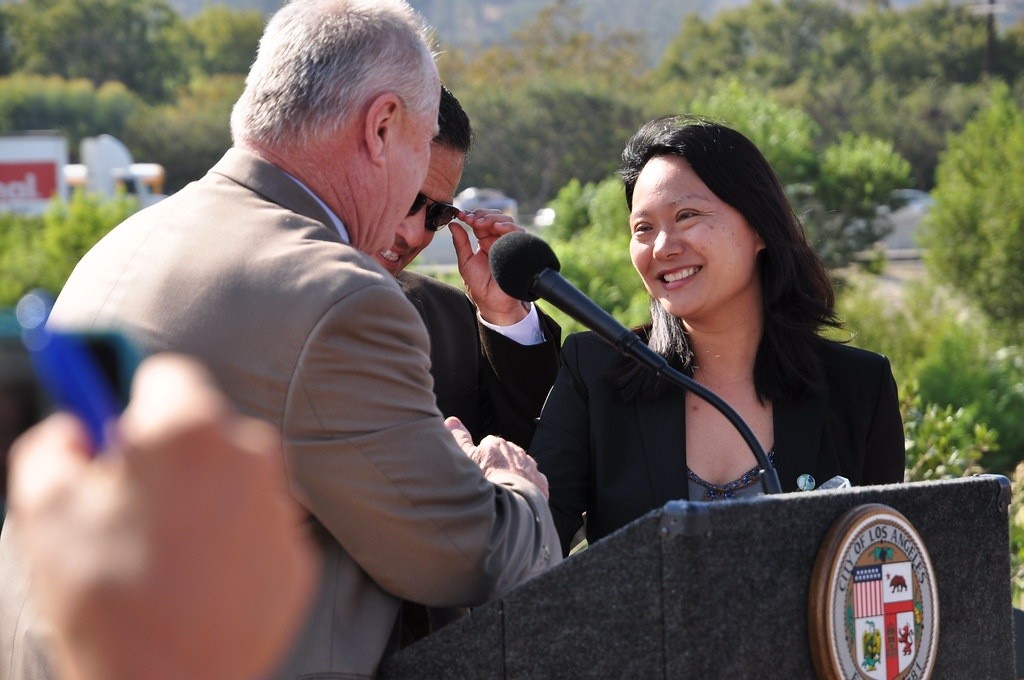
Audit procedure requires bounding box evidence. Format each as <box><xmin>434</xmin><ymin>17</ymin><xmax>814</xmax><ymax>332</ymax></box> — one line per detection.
<box><xmin>528</xmin><ymin>113</ymin><xmax>908</xmax><ymax>559</ymax></box>
<box><xmin>43</xmin><ymin>1</ymin><xmax>582</xmax><ymax>680</ymax></box>
<box><xmin>0</xmin><ymin>350</ymin><xmax>321</xmax><ymax>680</ymax></box>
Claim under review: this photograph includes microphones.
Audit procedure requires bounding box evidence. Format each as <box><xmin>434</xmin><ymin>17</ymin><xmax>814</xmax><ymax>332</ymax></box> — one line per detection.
<box><xmin>490</xmin><ymin>232</ymin><xmax>670</xmax><ymax>373</ymax></box>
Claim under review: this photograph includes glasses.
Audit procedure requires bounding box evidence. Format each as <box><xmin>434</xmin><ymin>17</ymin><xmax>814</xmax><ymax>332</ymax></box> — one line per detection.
<box><xmin>406</xmin><ymin>190</ymin><xmax>460</xmax><ymax>232</ymax></box>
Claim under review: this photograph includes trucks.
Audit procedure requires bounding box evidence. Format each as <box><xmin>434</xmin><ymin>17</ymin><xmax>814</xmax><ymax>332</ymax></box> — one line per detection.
<box><xmin>0</xmin><ymin>131</ymin><xmax>169</xmax><ymax>217</ymax></box>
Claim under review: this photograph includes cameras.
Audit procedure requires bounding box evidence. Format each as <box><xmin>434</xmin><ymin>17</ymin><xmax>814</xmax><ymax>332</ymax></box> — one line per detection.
<box><xmin>0</xmin><ymin>328</ymin><xmax>133</xmax><ymax>508</ymax></box>
